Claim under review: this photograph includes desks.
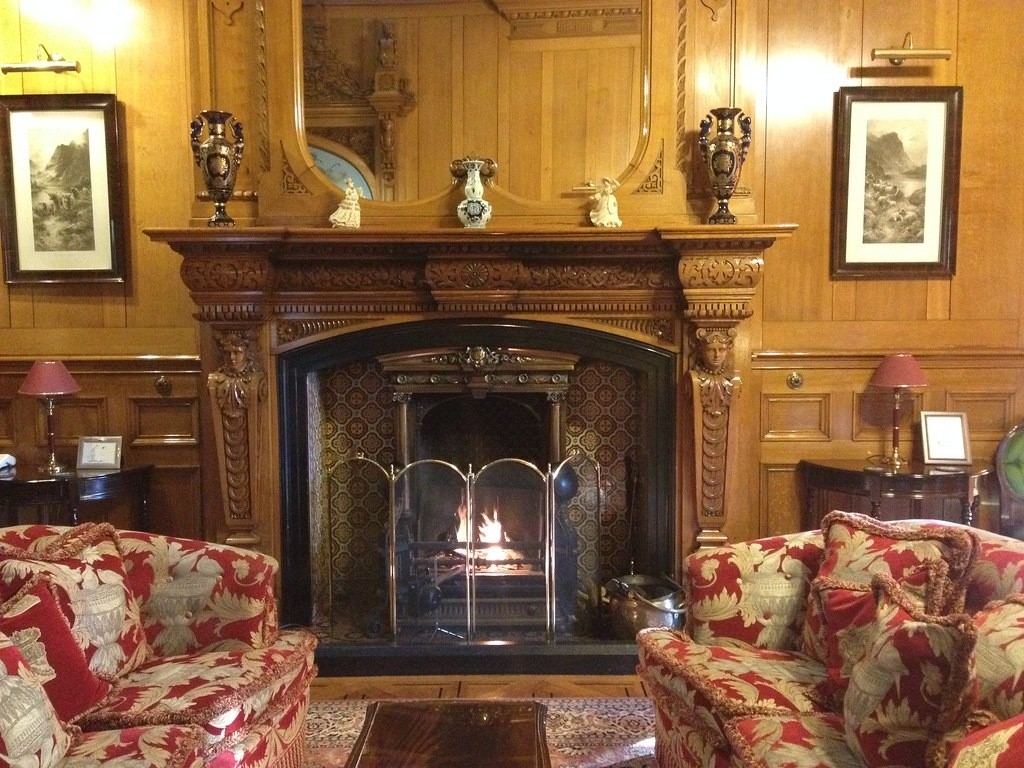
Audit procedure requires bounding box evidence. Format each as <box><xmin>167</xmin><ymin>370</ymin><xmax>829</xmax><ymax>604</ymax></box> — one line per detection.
<box><xmin>801</xmin><ymin>457</ymin><xmax>995</xmax><ymax>531</ymax></box>
<box><xmin>1</xmin><ymin>464</ymin><xmax>154</xmax><ymax>532</ymax></box>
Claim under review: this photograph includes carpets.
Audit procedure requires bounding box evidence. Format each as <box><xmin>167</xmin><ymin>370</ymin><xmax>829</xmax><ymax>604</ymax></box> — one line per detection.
<box><xmin>302</xmin><ymin>698</ymin><xmax>661</xmax><ymax>767</ymax></box>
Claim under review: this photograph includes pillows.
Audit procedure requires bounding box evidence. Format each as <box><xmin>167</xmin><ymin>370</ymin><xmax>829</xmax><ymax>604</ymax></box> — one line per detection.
<box><xmin>804</xmin><ymin>509</ymin><xmax>978</xmax><ymax>768</ymax></box>
<box><xmin>0</xmin><ymin>522</ymin><xmax>154</xmax><ymax>768</ymax></box>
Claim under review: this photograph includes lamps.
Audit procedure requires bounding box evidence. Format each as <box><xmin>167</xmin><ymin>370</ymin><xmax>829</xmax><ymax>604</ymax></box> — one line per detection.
<box><xmin>17</xmin><ymin>358</ymin><xmax>79</xmax><ymax>472</ymax></box>
<box><xmin>871</xmin><ymin>356</ymin><xmax>924</xmax><ymax>467</ymax></box>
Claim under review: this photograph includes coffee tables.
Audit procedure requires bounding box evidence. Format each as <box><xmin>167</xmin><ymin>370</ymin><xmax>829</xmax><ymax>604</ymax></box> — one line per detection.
<box><xmin>345</xmin><ymin>700</ymin><xmax>551</xmax><ymax>768</ymax></box>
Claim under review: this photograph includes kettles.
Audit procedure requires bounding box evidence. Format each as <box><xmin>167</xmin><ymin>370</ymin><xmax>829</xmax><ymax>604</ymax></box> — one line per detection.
<box><xmin>581</xmin><ymin>572</ymin><xmax>687</xmax><ymax>639</ymax></box>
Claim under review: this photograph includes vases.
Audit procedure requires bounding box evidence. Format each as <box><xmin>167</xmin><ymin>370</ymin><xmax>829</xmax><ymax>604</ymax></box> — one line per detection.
<box><xmin>189</xmin><ymin>110</ymin><xmax>245</xmax><ymax>229</ymax></box>
<box><xmin>457</xmin><ymin>162</ymin><xmax>491</xmax><ymax>229</ymax></box>
<box><xmin>698</xmin><ymin>107</ymin><xmax>752</xmax><ymax>225</ymax></box>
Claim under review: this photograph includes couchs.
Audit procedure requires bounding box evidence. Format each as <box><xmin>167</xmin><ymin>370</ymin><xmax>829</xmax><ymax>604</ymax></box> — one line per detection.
<box><xmin>636</xmin><ymin>518</ymin><xmax>1024</xmax><ymax>768</ymax></box>
<box><xmin>1</xmin><ymin>523</ymin><xmax>317</xmax><ymax>768</ymax></box>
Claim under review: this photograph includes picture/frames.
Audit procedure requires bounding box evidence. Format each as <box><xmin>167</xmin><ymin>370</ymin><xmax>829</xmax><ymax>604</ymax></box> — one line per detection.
<box><xmin>76</xmin><ymin>436</ymin><xmax>122</xmax><ymax>468</ymax></box>
<box><xmin>919</xmin><ymin>410</ymin><xmax>972</xmax><ymax>465</ymax></box>
<box><xmin>0</xmin><ymin>91</ymin><xmax>130</xmax><ymax>285</ymax></box>
<box><xmin>829</xmin><ymin>86</ymin><xmax>963</xmax><ymax>280</ymax></box>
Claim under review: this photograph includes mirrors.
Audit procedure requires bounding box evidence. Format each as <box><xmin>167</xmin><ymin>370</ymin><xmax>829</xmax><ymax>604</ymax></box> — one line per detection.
<box><xmin>263</xmin><ymin>1</ymin><xmax>656</xmax><ymax>203</ymax></box>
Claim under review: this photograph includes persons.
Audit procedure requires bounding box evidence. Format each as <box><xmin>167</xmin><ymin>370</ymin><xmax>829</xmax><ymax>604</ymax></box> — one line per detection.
<box><xmin>328</xmin><ymin>178</ymin><xmax>365</xmax><ymax>228</ymax></box>
<box><xmin>588</xmin><ymin>178</ymin><xmax>622</xmax><ymax>227</ymax></box>
